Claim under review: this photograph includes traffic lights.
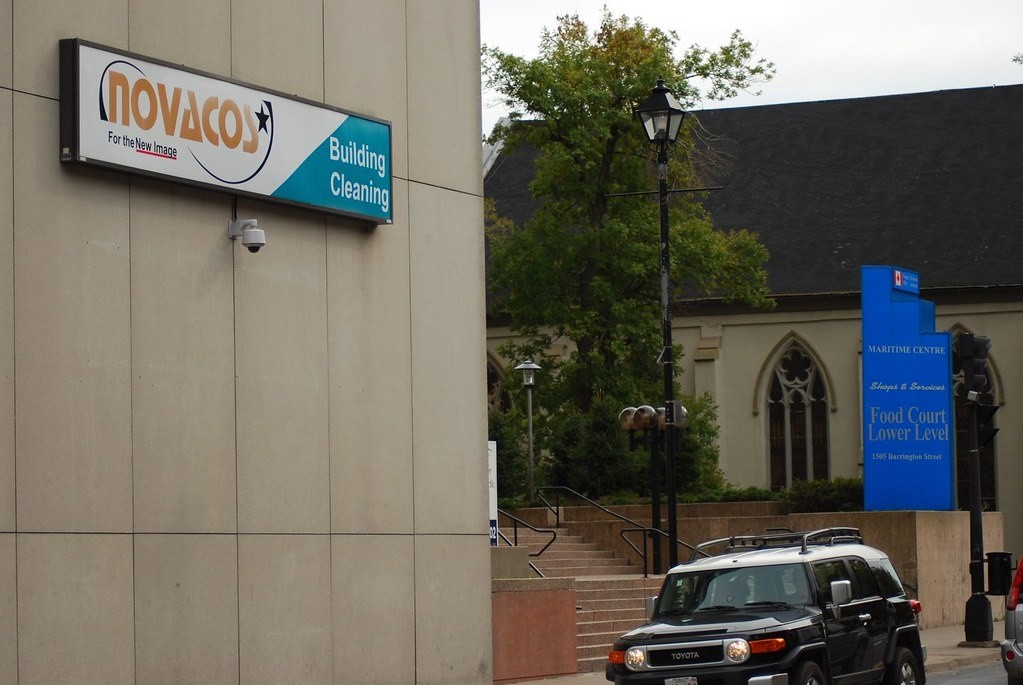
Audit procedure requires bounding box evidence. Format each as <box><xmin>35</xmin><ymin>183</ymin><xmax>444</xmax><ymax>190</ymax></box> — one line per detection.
<box><xmin>970</xmin><ymin>333</ymin><xmax>992</xmax><ymax>389</ymax></box>
<box><xmin>976</xmin><ymin>402</ymin><xmax>1000</xmax><ymax>446</ymax></box>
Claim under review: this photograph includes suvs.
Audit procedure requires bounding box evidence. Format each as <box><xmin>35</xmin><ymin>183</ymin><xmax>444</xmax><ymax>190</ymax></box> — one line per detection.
<box><xmin>605</xmin><ymin>527</ymin><xmax>927</xmax><ymax>685</ymax></box>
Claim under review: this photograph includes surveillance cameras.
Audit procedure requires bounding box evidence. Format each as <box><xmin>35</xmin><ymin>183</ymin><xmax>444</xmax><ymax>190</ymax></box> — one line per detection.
<box><xmin>241</xmin><ymin>228</ymin><xmax>266</xmax><ymax>253</ymax></box>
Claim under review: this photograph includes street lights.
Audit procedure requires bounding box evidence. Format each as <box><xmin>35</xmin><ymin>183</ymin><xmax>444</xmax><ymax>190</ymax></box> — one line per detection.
<box><xmin>636</xmin><ymin>74</ymin><xmax>688</xmax><ymax>564</ymax></box>
<box><xmin>618</xmin><ymin>404</ymin><xmax>688</xmax><ymax>574</ymax></box>
<box><xmin>514</xmin><ymin>360</ymin><xmax>543</xmax><ymax>508</ymax></box>
